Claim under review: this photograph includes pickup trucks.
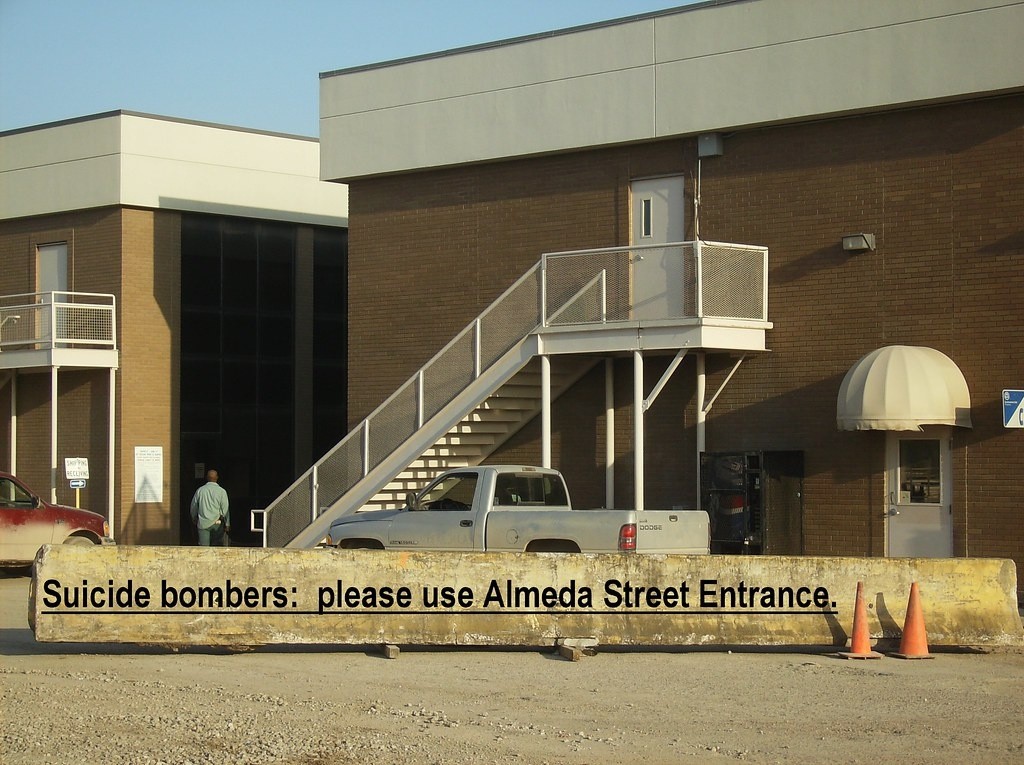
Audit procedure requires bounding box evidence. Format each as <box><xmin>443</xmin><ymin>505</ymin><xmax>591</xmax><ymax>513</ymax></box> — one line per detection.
<box><xmin>325</xmin><ymin>463</ymin><xmax>713</xmax><ymax>555</ymax></box>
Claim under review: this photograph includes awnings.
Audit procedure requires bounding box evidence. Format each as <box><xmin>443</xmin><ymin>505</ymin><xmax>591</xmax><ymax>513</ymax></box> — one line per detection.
<box><xmin>835</xmin><ymin>342</ymin><xmax>972</xmax><ymax>435</ymax></box>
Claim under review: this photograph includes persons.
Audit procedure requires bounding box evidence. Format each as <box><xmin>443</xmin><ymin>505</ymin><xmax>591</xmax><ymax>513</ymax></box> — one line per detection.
<box><xmin>188</xmin><ymin>469</ymin><xmax>231</xmax><ymax>547</ymax></box>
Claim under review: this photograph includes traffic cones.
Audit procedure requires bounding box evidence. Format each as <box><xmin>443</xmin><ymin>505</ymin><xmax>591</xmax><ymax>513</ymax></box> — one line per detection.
<box><xmin>837</xmin><ymin>579</ymin><xmax>885</xmax><ymax>660</ymax></box>
<box><xmin>887</xmin><ymin>583</ymin><xmax>934</xmax><ymax>659</ymax></box>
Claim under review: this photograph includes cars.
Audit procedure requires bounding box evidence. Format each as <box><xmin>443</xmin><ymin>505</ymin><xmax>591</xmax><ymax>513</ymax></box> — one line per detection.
<box><xmin>0</xmin><ymin>471</ymin><xmax>111</xmax><ymax>569</ymax></box>
